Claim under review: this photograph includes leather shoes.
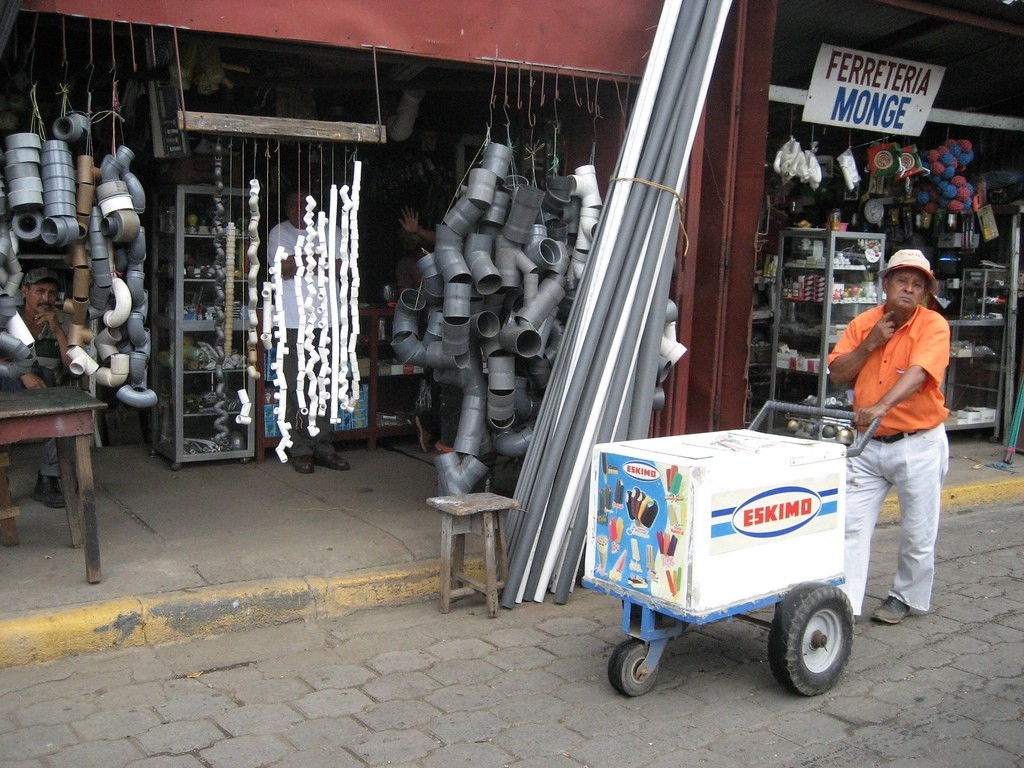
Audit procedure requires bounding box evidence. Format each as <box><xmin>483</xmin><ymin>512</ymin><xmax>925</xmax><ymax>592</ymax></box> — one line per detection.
<box><xmin>870</xmin><ymin>596</ymin><xmax>911</xmax><ymax>624</ymax></box>
<box><xmin>293</xmin><ymin>454</ymin><xmax>315</xmax><ymax>474</ymax></box>
<box><xmin>32</xmin><ymin>470</ymin><xmax>66</xmax><ymax>507</ymax></box>
<box><xmin>313</xmin><ymin>452</ymin><xmax>350</xmax><ymax>471</ymax></box>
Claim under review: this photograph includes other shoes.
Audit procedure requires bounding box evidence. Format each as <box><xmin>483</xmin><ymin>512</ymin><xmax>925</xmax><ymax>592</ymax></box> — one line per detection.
<box><xmin>414</xmin><ymin>415</ymin><xmax>434</xmax><ymax>454</ymax></box>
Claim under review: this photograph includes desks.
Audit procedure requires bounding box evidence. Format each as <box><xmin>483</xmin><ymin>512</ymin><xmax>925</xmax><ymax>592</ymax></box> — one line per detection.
<box><xmin>0</xmin><ymin>385</ymin><xmax>109</xmax><ymax>584</ymax></box>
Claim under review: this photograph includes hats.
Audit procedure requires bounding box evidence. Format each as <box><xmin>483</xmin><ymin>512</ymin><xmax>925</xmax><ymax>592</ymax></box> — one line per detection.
<box><xmin>21</xmin><ymin>266</ymin><xmax>62</xmax><ymax>290</ymax></box>
<box><xmin>876</xmin><ymin>248</ymin><xmax>939</xmax><ymax>295</ymax></box>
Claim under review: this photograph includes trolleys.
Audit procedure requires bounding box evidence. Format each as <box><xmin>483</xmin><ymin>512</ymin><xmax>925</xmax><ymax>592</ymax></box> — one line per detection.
<box><xmin>580</xmin><ymin>400</ymin><xmax>883</xmax><ymax>697</ymax></box>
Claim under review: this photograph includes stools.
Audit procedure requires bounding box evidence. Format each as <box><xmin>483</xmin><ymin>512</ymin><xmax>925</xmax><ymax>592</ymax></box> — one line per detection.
<box><xmin>425</xmin><ymin>492</ymin><xmax>519</xmax><ymax>618</ymax></box>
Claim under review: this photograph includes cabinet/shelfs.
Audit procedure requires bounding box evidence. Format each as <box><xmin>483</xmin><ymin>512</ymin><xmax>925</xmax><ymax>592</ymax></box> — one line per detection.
<box><xmin>149</xmin><ymin>183</ymin><xmax>256</xmax><ymax>471</ymax></box>
<box><xmin>749</xmin><ymin>228</ymin><xmax>887</xmax><ymax>445</ymax></box>
<box><xmin>256</xmin><ymin>306</ymin><xmax>428</xmax><ymax>465</ymax></box>
<box><xmin>958</xmin><ymin>267</ymin><xmax>1011</xmax><ymax>322</ymax></box>
<box><xmin>944</xmin><ymin>320</ymin><xmax>1009</xmax><ymax>445</ymax></box>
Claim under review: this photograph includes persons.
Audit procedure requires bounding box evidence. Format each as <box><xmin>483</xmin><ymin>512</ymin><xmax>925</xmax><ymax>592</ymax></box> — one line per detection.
<box><xmin>267</xmin><ymin>186</ymin><xmax>352</xmax><ymax>472</ymax></box>
<box><xmin>392</xmin><ymin>203</ymin><xmax>460</xmax><ymax>455</ymax></box>
<box><xmin>828</xmin><ymin>249</ymin><xmax>950</xmax><ymax>625</ymax></box>
<box><xmin>0</xmin><ymin>266</ymin><xmax>80</xmax><ymax>511</ymax></box>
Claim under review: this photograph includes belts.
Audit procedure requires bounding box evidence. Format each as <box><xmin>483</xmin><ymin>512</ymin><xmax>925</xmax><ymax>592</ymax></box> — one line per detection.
<box><xmin>871</xmin><ymin>430</ymin><xmax>917</xmax><ymax>444</ymax></box>
